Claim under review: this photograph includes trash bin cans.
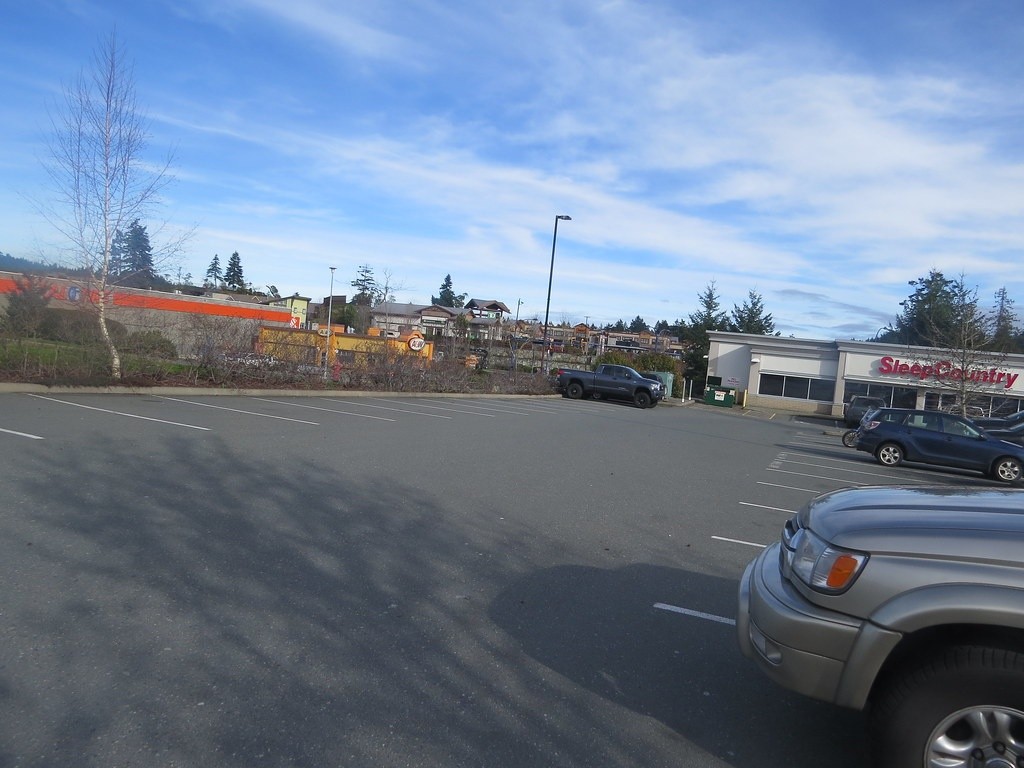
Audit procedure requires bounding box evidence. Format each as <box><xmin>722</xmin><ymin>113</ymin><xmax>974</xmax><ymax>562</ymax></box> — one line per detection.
<box><xmin>705</xmin><ymin>385</ymin><xmax>735</xmax><ymax>408</ymax></box>
<box><xmin>637</xmin><ymin>370</ymin><xmax>674</xmax><ymax>401</ymax></box>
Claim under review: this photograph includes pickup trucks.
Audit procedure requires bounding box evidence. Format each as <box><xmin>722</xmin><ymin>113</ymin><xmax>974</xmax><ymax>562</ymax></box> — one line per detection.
<box><xmin>552</xmin><ymin>363</ymin><xmax>668</xmax><ymax>409</ymax></box>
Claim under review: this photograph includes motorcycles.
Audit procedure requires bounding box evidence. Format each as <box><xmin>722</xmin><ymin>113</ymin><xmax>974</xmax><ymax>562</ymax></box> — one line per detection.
<box><xmin>842</xmin><ymin>405</ymin><xmax>882</xmax><ymax>448</ymax></box>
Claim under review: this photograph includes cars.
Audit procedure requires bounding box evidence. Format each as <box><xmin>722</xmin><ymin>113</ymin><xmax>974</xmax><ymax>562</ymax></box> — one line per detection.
<box><xmin>843</xmin><ymin>395</ymin><xmax>887</xmax><ymax>430</ymax></box>
<box><xmin>591</xmin><ymin>373</ymin><xmax>665</xmax><ymax>403</ymax></box>
<box><xmin>966</xmin><ymin>409</ymin><xmax>1024</xmax><ymax>446</ymax></box>
<box><xmin>856</xmin><ymin>407</ymin><xmax>1024</xmax><ymax>483</ymax></box>
<box><xmin>737</xmin><ymin>483</ymin><xmax>1023</xmax><ymax>768</ymax></box>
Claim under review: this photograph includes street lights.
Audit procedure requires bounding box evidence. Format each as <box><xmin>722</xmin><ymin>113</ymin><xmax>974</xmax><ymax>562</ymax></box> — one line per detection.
<box><xmin>324</xmin><ymin>265</ymin><xmax>337</xmax><ymax>379</ymax></box>
<box><xmin>516</xmin><ymin>298</ymin><xmax>524</xmax><ymax>321</ymax></box>
<box><xmin>539</xmin><ymin>215</ymin><xmax>572</xmax><ymax>375</ymax></box>
